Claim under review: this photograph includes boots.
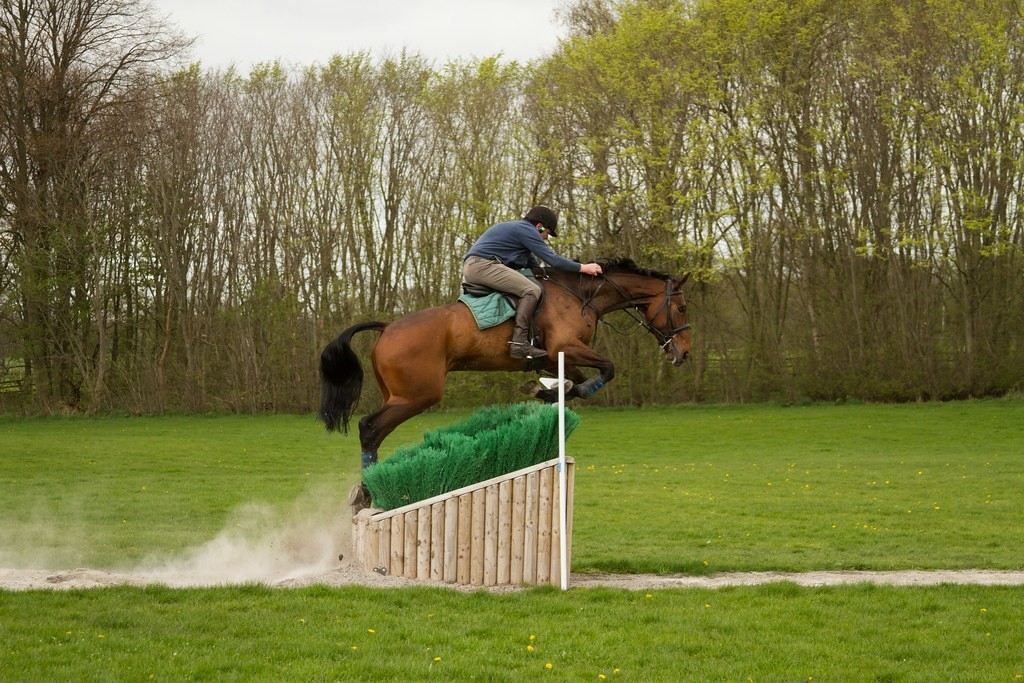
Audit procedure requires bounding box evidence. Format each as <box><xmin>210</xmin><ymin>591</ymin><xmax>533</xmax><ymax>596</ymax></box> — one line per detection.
<box><xmin>510</xmin><ymin>290</ymin><xmax>548</xmax><ymax>358</ymax></box>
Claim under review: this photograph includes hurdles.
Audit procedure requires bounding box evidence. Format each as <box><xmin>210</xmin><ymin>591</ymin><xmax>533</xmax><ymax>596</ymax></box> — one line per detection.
<box><xmin>350</xmin><ymin>349</ymin><xmax>576</xmax><ymax>595</ymax></box>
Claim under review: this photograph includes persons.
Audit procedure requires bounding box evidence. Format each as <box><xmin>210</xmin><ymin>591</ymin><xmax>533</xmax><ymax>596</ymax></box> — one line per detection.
<box><xmin>462</xmin><ymin>206</ymin><xmax>603</xmax><ymax>360</ymax></box>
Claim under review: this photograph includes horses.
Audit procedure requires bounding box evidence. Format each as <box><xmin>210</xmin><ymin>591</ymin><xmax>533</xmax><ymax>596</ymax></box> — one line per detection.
<box><xmin>319</xmin><ymin>256</ymin><xmax>692</xmax><ymax>515</ymax></box>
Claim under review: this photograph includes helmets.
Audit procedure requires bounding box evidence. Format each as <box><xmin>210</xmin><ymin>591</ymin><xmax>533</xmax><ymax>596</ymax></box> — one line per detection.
<box><xmin>525</xmin><ymin>207</ymin><xmax>557</xmax><ymax>237</ymax></box>
